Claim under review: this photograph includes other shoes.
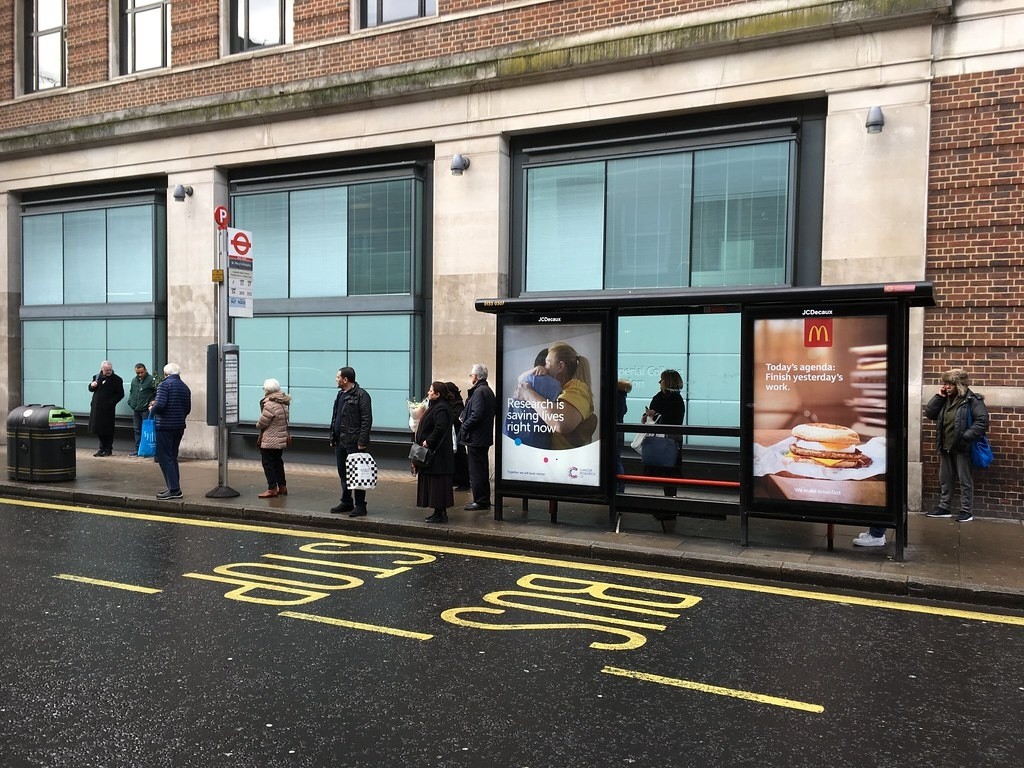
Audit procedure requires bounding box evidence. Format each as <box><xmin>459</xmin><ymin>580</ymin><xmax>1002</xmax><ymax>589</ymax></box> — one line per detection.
<box><xmin>258</xmin><ymin>487</ymin><xmax>278</xmax><ymax>498</ymax></box>
<box><xmin>330</xmin><ymin>499</ymin><xmax>354</xmax><ymax>513</ymax></box>
<box><xmin>278</xmin><ymin>486</ymin><xmax>287</xmax><ymax>495</ymax></box>
<box><xmin>425</xmin><ymin>513</ymin><xmax>448</xmax><ymax>523</ymax></box>
<box><xmin>93</xmin><ymin>449</ymin><xmax>112</xmax><ymax>457</ymax></box>
<box><xmin>455</xmin><ymin>486</ymin><xmax>472</xmax><ymax>491</ymax></box>
<box><xmin>349</xmin><ymin>502</ymin><xmax>367</xmax><ymax>517</ymax></box>
<box><xmin>128</xmin><ymin>451</ymin><xmax>138</xmax><ymax>458</ymax></box>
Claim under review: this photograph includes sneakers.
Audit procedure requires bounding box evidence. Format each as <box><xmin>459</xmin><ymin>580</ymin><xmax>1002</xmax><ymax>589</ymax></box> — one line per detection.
<box><xmin>926</xmin><ymin>506</ymin><xmax>953</xmax><ymax>518</ymax></box>
<box><xmin>853</xmin><ymin>534</ymin><xmax>885</xmax><ymax>547</ymax></box>
<box><xmin>156</xmin><ymin>489</ymin><xmax>183</xmax><ymax>500</ymax></box>
<box><xmin>858</xmin><ymin>532</ymin><xmax>886</xmax><ymax>543</ymax></box>
<box><xmin>955</xmin><ymin>511</ymin><xmax>973</xmax><ymax>522</ymax></box>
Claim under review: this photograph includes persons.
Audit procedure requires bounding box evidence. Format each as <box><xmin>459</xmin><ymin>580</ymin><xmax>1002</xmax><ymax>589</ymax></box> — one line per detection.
<box><xmin>329</xmin><ymin>366</ymin><xmax>373</xmax><ymax>518</ymax></box>
<box><xmin>641</xmin><ymin>370</ymin><xmax>685</xmax><ymax>497</ymax></box>
<box><xmin>87</xmin><ymin>361</ymin><xmax>125</xmax><ymax>457</ymax></box>
<box><xmin>852</xmin><ymin>527</ymin><xmax>886</xmax><ymax>546</ymax></box>
<box><xmin>148</xmin><ymin>363</ymin><xmax>191</xmax><ymax>499</ymax></box>
<box><xmin>410</xmin><ymin>380</ymin><xmax>454</xmax><ymax>523</ymax></box>
<box><xmin>617</xmin><ymin>380</ymin><xmax>633</xmax><ymax>494</ymax></box>
<box><xmin>127</xmin><ymin>363</ymin><xmax>154</xmax><ymax>456</ymax></box>
<box><xmin>256</xmin><ymin>378</ymin><xmax>291</xmax><ymax>499</ymax></box>
<box><xmin>445</xmin><ymin>382</ymin><xmax>471</xmax><ymax>491</ymax></box>
<box><xmin>924</xmin><ymin>369</ymin><xmax>987</xmax><ymax>522</ymax></box>
<box><xmin>503</xmin><ymin>341</ymin><xmax>597</xmax><ymax>451</ymax></box>
<box><xmin>456</xmin><ymin>364</ymin><xmax>495</xmax><ymax>510</ymax></box>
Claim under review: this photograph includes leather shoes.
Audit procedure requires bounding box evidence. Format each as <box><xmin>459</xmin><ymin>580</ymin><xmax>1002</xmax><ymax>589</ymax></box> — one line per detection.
<box><xmin>463</xmin><ymin>502</ymin><xmax>489</xmax><ymax>510</ymax></box>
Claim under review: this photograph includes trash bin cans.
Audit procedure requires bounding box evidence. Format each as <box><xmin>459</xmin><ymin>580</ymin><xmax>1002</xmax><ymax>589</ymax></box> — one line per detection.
<box><xmin>6</xmin><ymin>403</ymin><xmax>76</xmax><ymax>483</ymax></box>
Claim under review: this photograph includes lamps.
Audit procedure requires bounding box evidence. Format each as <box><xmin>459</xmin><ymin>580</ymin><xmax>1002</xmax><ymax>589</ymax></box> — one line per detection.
<box><xmin>865</xmin><ymin>107</ymin><xmax>884</xmax><ymax>133</ymax></box>
<box><xmin>450</xmin><ymin>154</ymin><xmax>470</xmax><ymax>175</ymax></box>
<box><xmin>173</xmin><ymin>184</ymin><xmax>193</xmax><ymax>202</ymax></box>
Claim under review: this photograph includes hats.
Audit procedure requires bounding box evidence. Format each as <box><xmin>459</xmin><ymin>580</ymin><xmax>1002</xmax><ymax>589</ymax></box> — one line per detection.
<box><xmin>941</xmin><ymin>369</ymin><xmax>969</xmax><ymax>398</ymax></box>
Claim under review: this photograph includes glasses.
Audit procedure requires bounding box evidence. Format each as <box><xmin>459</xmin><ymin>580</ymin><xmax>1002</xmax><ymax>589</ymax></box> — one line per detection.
<box><xmin>470</xmin><ymin>374</ymin><xmax>474</xmax><ymax>377</ymax></box>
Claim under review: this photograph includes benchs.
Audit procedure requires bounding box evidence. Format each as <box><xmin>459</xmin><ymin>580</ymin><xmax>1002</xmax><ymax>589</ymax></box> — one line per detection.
<box><xmin>616</xmin><ymin>473</ymin><xmax>741</xmax><ymax>522</ymax></box>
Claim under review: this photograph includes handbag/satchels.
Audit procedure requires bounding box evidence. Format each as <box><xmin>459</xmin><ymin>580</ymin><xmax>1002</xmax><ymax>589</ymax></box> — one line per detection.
<box><xmin>408</xmin><ymin>443</ymin><xmax>433</xmax><ymax>465</ymax></box>
<box><xmin>345</xmin><ymin>447</ymin><xmax>377</xmax><ymax>491</ymax></box>
<box><xmin>138</xmin><ymin>410</ymin><xmax>157</xmax><ymax>457</ymax></box>
<box><xmin>641</xmin><ymin>416</ymin><xmax>680</xmax><ymax>475</ymax></box>
<box><xmin>972</xmin><ymin>435</ymin><xmax>993</xmax><ymax>469</ymax></box>
<box><xmin>631</xmin><ymin>413</ymin><xmax>666</xmax><ymax>455</ymax></box>
<box><xmin>286</xmin><ymin>435</ymin><xmax>292</xmax><ymax>447</ymax></box>
